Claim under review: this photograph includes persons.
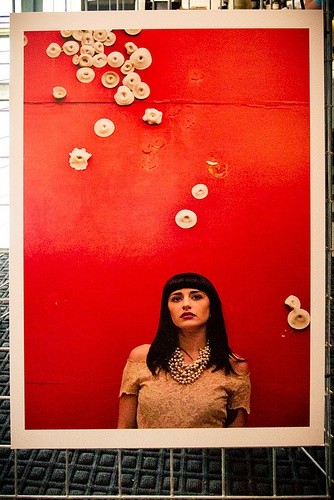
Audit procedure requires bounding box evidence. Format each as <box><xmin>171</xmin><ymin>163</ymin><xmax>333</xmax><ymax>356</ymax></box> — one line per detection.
<box><xmin>117</xmin><ymin>273</ymin><xmax>250</xmax><ymax>428</ymax></box>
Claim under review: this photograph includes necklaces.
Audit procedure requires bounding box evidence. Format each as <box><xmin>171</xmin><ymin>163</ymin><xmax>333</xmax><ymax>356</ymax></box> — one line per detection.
<box><xmin>167</xmin><ymin>340</ymin><xmax>211</xmax><ymax>385</ymax></box>
